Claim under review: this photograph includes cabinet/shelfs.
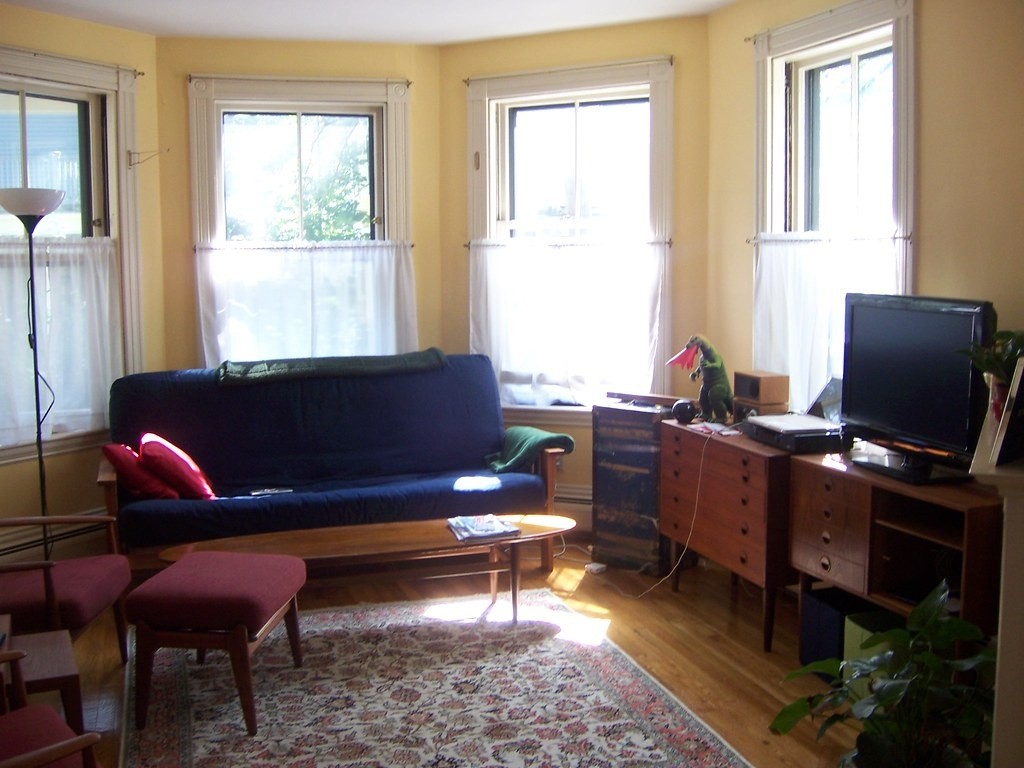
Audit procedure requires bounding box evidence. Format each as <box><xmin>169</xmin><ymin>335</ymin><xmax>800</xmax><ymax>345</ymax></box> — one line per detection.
<box><xmin>787</xmin><ymin>453</ymin><xmax>1004</xmax><ymax>690</ymax></box>
<box><xmin>658</xmin><ymin>421</ymin><xmax>793</xmax><ymax>651</ymax></box>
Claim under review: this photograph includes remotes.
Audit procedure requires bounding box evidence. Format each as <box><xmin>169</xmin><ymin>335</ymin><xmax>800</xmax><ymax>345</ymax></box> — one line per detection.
<box><xmin>250</xmin><ymin>486</ymin><xmax>293</xmax><ymax>496</ymax></box>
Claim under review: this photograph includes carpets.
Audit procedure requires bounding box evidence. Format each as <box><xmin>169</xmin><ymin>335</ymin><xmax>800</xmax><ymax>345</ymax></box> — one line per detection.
<box><xmin>121</xmin><ymin>587</ymin><xmax>753</xmax><ymax>768</ymax></box>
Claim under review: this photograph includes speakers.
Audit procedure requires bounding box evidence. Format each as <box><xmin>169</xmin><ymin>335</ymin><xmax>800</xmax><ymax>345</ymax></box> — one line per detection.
<box><xmin>732</xmin><ymin>371</ymin><xmax>789</xmax><ymax>433</ymax></box>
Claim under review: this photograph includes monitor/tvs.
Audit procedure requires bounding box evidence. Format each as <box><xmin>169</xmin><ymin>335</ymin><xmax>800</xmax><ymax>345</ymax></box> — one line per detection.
<box><xmin>840</xmin><ymin>293</ymin><xmax>997</xmax><ymax>485</ymax></box>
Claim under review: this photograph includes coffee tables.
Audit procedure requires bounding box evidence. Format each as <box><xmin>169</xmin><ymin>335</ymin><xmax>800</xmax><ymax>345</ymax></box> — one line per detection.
<box><xmin>161</xmin><ymin>514</ymin><xmax>576</xmax><ymax>625</ymax></box>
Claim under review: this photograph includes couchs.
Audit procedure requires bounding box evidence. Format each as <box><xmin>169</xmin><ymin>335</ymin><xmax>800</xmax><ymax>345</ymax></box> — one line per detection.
<box><xmin>92</xmin><ymin>347</ymin><xmax>563</xmax><ymax>573</ymax></box>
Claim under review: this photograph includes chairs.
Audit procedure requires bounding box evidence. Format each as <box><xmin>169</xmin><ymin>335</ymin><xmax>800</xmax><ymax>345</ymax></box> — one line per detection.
<box><xmin>0</xmin><ymin>651</ymin><xmax>100</xmax><ymax>768</ymax></box>
<box><xmin>0</xmin><ymin>515</ymin><xmax>130</xmax><ymax>662</ymax></box>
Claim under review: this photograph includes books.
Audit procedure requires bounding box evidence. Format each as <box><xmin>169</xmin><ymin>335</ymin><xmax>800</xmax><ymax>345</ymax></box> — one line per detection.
<box><xmin>448</xmin><ymin>513</ymin><xmax>521</xmax><ymax>543</ymax></box>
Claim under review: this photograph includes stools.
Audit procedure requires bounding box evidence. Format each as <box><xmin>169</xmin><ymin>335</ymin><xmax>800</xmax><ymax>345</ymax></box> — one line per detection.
<box><xmin>126</xmin><ymin>550</ymin><xmax>305</xmax><ymax>735</ymax></box>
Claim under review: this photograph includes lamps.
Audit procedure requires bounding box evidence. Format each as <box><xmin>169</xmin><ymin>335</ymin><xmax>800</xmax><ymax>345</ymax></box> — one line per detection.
<box><xmin>0</xmin><ymin>186</ymin><xmax>66</xmax><ymax>235</ymax></box>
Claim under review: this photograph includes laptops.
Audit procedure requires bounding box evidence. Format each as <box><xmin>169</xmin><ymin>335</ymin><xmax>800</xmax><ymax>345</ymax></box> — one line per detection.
<box><xmin>747</xmin><ymin>377</ymin><xmax>842</xmax><ymax>435</ymax></box>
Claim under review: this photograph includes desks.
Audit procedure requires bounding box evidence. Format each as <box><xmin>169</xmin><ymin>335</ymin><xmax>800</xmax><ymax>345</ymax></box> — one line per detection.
<box><xmin>0</xmin><ymin>629</ymin><xmax>84</xmax><ymax>734</ymax></box>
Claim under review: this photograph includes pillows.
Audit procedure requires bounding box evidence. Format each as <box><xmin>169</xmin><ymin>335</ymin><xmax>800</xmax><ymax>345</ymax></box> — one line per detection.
<box><xmin>138</xmin><ymin>430</ymin><xmax>215</xmax><ymax>500</ymax></box>
<box><xmin>101</xmin><ymin>443</ymin><xmax>179</xmax><ymax>501</ymax></box>
